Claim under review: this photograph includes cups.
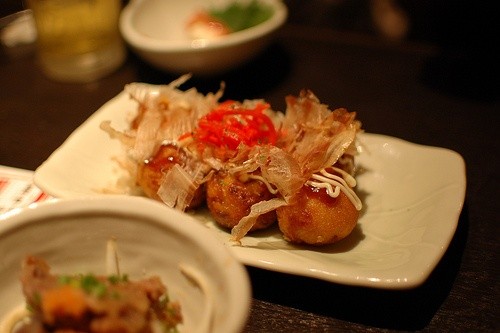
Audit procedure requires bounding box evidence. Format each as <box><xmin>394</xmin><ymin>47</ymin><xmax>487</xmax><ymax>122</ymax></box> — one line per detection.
<box><xmin>28</xmin><ymin>0</ymin><xmax>129</xmax><ymax>82</ymax></box>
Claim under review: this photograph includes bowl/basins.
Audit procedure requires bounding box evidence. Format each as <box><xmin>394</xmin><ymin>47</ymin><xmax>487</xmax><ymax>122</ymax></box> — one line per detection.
<box><xmin>0</xmin><ymin>194</ymin><xmax>253</xmax><ymax>332</ymax></box>
<box><xmin>118</xmin><ymin>1</ymin><xmax>289</xmax><ymax>79</ymax></box>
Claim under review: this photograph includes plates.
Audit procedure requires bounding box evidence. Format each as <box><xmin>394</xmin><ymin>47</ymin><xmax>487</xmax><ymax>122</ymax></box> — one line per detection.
<box><xmin>37</xmin><ymin>83</ymin><xmax>468</xmax><ymax>292</ymax></box>
